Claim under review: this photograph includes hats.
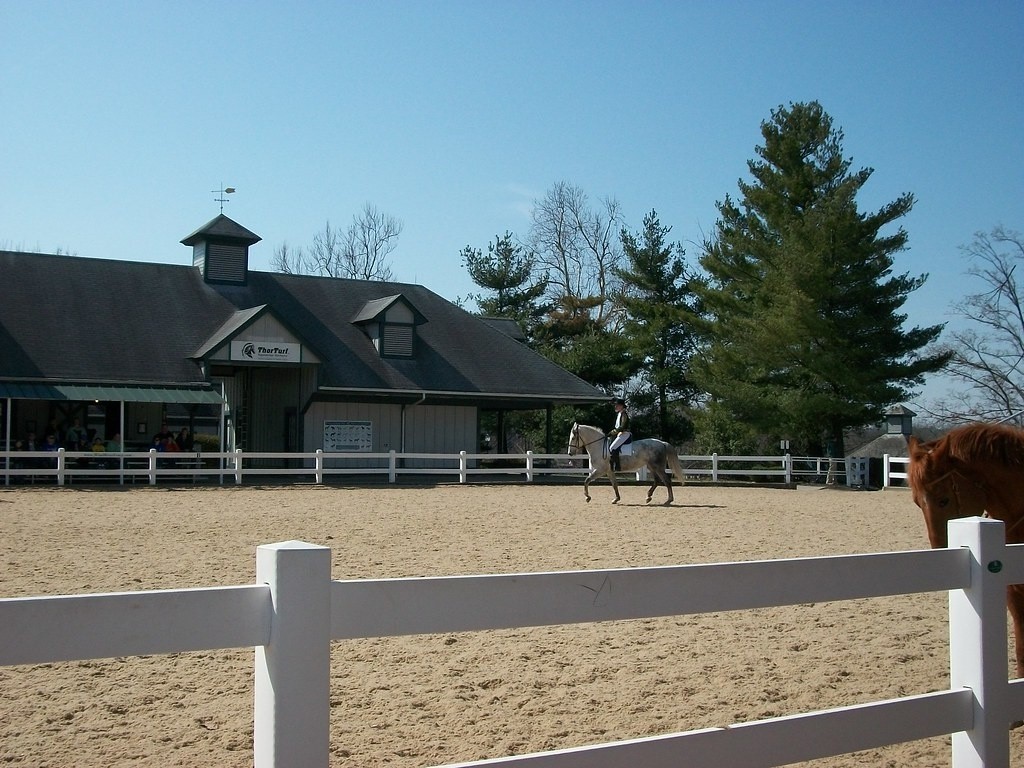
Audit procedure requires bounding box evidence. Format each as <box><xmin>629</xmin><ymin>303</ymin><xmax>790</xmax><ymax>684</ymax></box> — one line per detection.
<box><xmin>614</xmin><ymin>399</ymin><xmax>625</xmax><ymax>404</ymax></box>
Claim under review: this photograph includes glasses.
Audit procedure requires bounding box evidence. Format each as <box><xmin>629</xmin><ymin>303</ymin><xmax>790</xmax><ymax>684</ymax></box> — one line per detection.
<box><xmin>615</xmin><ymin>405</ymin><xmax>619</xmax><ymax>408</ymax></box>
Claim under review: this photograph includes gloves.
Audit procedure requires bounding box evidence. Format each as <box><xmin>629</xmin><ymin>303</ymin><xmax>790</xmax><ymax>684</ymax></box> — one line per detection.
<box><xmin>608</xmin><ymin>429</ymin><xmax>618</xmax><ymax>435</ymax></box>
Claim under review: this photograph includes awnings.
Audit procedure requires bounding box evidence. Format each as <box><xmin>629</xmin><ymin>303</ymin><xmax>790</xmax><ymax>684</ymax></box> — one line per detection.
<box><xmin>0</xmin><ymin>380</ymin><xmax>226</xmax><ymax>404</ymax></box>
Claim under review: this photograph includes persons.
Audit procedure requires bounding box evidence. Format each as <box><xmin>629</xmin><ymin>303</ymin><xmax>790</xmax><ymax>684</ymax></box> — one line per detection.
<box><xmin>146</xmin><ymin>424</ymin><xmax>194</xmax><ymax>468</ymax></box>
<box><xmin>607</xmin><ymin>399</ymin><xmax>632</xmax><ymax>470</ymax></box>
<box><xmin>14</xmin><ymin>418</ymin><xmax>127</xmax><ymax>469</ymax></box>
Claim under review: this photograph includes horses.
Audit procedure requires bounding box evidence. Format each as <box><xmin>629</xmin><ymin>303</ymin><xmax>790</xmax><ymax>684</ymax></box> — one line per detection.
<box><xmin>909</xmin><ymin>424</ymin><xmax>1024</xmax><ymax>680</ymax></box>
<box><xmin>568</xmin><ymin>422</ymin><xmax>685</xmax><ymax>506</ymax></box>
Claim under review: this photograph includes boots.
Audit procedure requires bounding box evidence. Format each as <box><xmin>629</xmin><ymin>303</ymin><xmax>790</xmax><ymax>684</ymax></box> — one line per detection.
<box><xmin>610</xmin><ymin>449</ymin><xmax>622</xmax><ymax>471</ymax></box>
